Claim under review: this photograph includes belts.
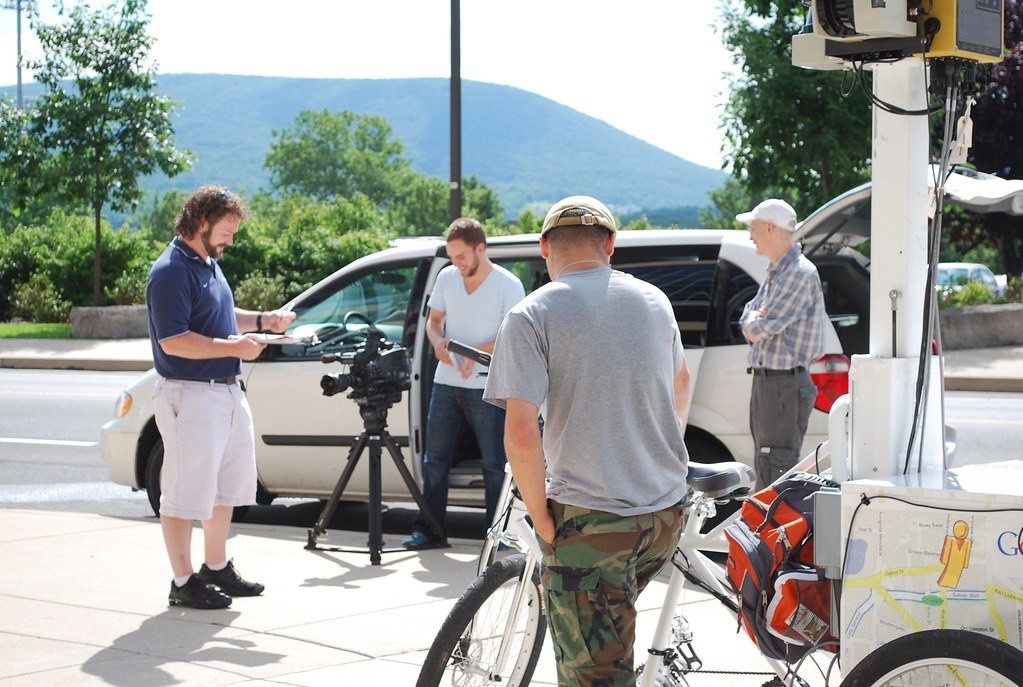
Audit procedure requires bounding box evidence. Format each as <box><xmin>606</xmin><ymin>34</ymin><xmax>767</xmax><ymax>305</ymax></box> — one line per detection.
<box><xmin>167</xmin><ymin>375</ymin><xmax>236</xmax><ymax>385</ymax></box>
<box><xmin>747</xmin><ymin>367</ymin><xmax>805</xmax><ymax>376</ymax></box>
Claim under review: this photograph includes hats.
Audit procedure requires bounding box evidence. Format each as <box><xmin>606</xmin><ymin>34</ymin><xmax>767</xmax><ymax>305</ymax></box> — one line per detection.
<box><xmin>736</xmin><ymin>198</ymin><xmax>796</xmax><ymax>231</ymax></box>
<box><xmin>542</xmin><ymin>195</ymin><xmax>617</xmax><ymax>235</ymax></box>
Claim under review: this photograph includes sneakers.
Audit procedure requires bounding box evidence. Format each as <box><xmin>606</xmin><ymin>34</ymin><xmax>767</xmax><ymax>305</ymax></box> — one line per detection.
<box><xmin>402</xmin><ymin>531</ymin><xmax>436</xmax><ymax>548</ymax></box>
<box><xmin>169</xmin><ymin>572</ymin><xmax>233</xmax><ymax>610</ymax></box>
<box><xmin>199</xmin><ymin>560</ymin><xmax>264</xmax><ymax>596</ymax></box>
<box><xmin>497</xmin><ymin>542</ymin><xmax>507</xmax><ymax>550</ymax></box>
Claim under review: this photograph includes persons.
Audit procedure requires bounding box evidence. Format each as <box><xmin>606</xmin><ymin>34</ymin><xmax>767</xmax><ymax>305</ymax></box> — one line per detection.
<box><xmin>144</xmin><ymin>185</ymin><xmax>295</xmax><ymax>609</ymax></box>
<box><xmin>402</xmin><ymin>217</ymin><xmax>526</xmax><ymax>550</ymax></box>
<box><xmin>482</xmin><ymin>196</ymin><xmax>691</xmax><ymax>687</ymax></box>
<box><xmin>734</xmin><ymin>199</ymin><xmax>826</xmax><ymax>497</ymax></box>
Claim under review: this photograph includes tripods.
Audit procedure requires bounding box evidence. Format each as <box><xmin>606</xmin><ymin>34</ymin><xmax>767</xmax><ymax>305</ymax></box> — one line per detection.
<box><xmin>304</xmin><ymin>404</ymin><xmax>451</xmax><ymax>565</ymax></box>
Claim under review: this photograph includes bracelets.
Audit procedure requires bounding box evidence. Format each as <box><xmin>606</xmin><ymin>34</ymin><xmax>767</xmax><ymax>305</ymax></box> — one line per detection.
<box><xmin>256</xmin><ymin>311</ymin><xmax>264</xmax><ymax>332</ymax></box>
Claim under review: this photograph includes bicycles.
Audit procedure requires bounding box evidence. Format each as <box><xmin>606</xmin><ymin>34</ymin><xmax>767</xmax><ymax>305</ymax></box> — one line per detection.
<box><xmin>414</xmin><ymin>342</ymin><xmax>1022</xmax><ymax>687</ymax></box>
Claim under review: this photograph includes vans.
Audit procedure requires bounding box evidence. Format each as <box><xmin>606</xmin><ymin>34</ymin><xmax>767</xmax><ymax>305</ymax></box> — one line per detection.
<box><xmin>95</xmin><ymin>230</ymin><xmax>957</xmax><ymax>558</ymax></box>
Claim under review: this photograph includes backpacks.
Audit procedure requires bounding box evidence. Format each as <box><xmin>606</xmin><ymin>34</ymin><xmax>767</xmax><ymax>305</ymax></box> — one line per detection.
<box><xmin>723</xmin><ymin>471</ymin><xmax>841</xmax><ymax>665</ymax></box>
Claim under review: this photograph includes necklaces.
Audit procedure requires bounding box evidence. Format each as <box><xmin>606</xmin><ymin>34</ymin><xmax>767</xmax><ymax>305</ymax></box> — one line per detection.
<box><xmin>555</xmin><ymin>260</ymin><xmax>601</xmax><ymax>278</ymax></box>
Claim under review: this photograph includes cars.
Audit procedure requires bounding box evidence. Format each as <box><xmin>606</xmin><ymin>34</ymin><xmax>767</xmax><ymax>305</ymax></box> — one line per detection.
<box><xmin>928</xmin><ymin>260</ymin><xmax>1006</xmax><ymax>301</ymax></box>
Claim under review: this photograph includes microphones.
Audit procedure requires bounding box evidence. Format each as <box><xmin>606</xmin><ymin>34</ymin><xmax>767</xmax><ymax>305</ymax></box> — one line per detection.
<box><xmin>321</xmin><ymin>353</ymin><xmax>336</xmax><ymax>364</ymax></box>
